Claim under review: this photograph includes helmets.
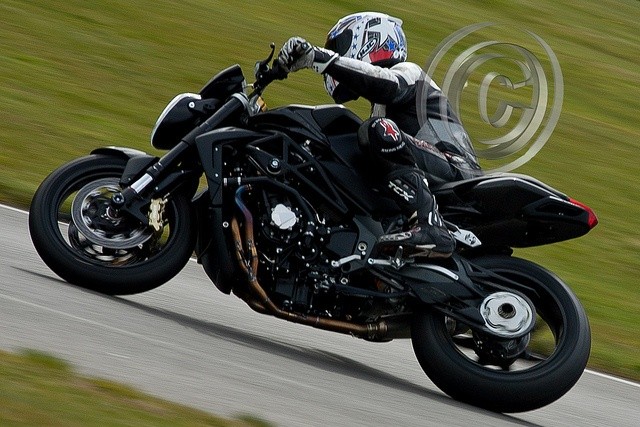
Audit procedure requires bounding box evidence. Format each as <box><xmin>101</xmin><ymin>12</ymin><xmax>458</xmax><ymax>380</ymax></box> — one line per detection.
<box><xmin>322</xmin><ymin>11</ymin><xmax>407</xmax><ymax>104</ymax></box>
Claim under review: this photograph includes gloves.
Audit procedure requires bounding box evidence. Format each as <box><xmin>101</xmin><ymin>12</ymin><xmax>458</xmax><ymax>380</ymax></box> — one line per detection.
<box><xmin>279</xmin><ymin>36</ymin><xmax>339</xmax><ymax>75</ymax></box>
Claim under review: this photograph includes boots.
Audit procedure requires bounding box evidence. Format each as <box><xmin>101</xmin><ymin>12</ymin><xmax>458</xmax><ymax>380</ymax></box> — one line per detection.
<box><xmin>379</xmin><ymin>167</ymin><xmax>456</xmax><ymax>260</ymax></box>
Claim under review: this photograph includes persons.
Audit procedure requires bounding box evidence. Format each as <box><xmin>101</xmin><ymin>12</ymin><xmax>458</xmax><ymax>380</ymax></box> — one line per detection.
<box><xmin>279</xmin><ymin>11</ymin><xmax>483</xmax><ymax>258</ymax></box>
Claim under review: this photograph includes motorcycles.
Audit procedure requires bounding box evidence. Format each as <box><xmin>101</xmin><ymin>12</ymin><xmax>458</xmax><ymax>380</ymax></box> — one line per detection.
<box><xmin>29</xmin><ymin>42</ymin><xmax>599</xmax><ymax>413</ymax></box>
